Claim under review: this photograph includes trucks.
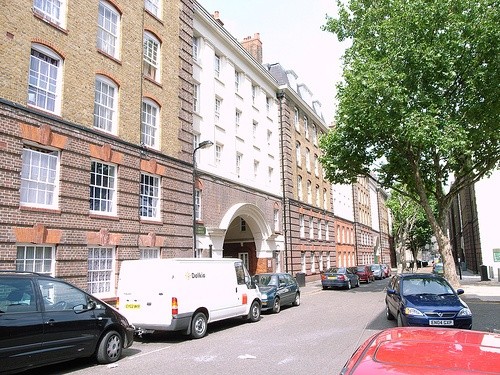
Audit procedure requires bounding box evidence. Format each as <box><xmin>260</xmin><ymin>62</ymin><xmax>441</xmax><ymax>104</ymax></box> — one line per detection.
<box><xmin>115</xmin><ymin>255</ymin><xmax>263</xmax><ymax>342</ymax></box>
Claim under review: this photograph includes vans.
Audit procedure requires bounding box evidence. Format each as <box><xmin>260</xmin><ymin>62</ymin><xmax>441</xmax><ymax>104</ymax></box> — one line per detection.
<box><xmin>0</xmin><ymin>268</ymin><xmax>141</xmax><ymax>375</ymax></box>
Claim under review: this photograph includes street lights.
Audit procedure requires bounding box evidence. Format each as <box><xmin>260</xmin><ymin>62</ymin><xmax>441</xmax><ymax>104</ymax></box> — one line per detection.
<box><xmin>191</xmin><ymin>139</ymin><xmax>215</xmax><ymax>258</ymax></box>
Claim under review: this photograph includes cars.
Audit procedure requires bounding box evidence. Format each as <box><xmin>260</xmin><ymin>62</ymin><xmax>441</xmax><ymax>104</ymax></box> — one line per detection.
<box><xmin>320</xmin><ymin>266</ymin><xmax>361</xmax><ymax>290</ymax></box>
<box><xmin>339</xmin><ymin>326</ymin><xmax>500</xmax><ymax>375</ymax></box>
<box><xmin>379</xmin><ymin>263</ymin><xmax>392</xmax><ymax>277</ymax></box>
<box><xmin>368</xmin><ymin>264</ymin><xmax>386</xmax><ymax>280</ymax></box>
<box><xmin>348</xmin><ymin>265</ymin><xmax>376</xmax><ymax>283</ymax></box>
<box><xmin>403</xmin><ymin>260</ymin><xmax>428</xmax><ymax>268</ymax></box>
<box><xmin>384</xmin><ymin>272</ymin><xmax>473</xmax><ymax>330</ymax></box>
<box><xmin>251</xmin><ymin>273</ymin><xmax>301</xmax><ymax>313</ymax></box>
